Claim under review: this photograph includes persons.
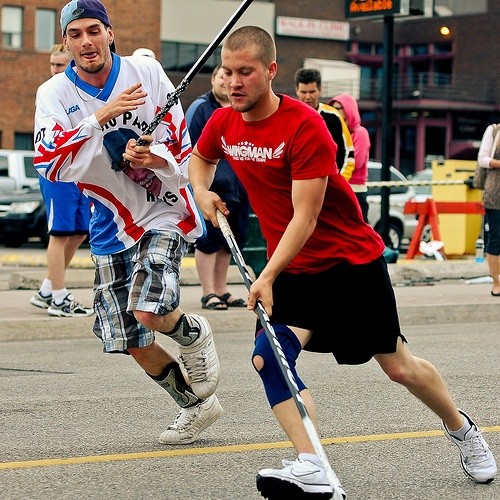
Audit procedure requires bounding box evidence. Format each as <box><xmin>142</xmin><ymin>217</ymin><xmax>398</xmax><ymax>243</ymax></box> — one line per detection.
<box><xmin>29</xmin><ymin>43</ymin><xmax>379</xmax><ymax>317</ymax></box>
<box><xmin>187</xmin><ymin>27</ymin><xmax>497</xmax><ymax>500</ymax></box>
<box><xmin>34</xmin><ymin>0</ymin><xmax>224</xmax><ymax>448</ymax></box>
<box><xmin>473</xmin><ymin>124</ymin><xmax>500</xmax><ymax>296</ymax></box>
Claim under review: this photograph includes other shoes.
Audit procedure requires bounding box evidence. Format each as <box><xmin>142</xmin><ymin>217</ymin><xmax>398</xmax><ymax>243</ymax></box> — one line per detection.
<box><xmin>491</xmin><ymin>286</ymin><xmax>500</xmax><ymax>296</ymax></box>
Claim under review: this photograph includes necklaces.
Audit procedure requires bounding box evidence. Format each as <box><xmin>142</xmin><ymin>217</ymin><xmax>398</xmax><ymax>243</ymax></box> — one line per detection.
<box><xmin>75</xmin><ymin>70</ymin><xmax>103</xmax><ymax>102</ymax></box>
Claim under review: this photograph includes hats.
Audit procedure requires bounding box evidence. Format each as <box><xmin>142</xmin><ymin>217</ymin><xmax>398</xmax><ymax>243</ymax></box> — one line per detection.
<box><xmin>132</xmin><ymin>47</ymin><xmax>156</xmax><ymax>59</ymax></box>
<box><xmin>60</xmin><ymin>0</ymin><xmax>114</xmax><ymax>38</ymax></box>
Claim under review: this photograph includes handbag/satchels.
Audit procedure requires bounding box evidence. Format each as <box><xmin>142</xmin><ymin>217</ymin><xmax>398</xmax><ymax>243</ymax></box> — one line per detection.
<box><xmin>472</xmin><ymin>123</ymin><xmax>496</xmax><ymax>190</ymax></box>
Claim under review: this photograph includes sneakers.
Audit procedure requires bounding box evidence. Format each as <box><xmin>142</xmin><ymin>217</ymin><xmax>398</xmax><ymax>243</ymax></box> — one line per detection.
<box><xmin>29</xmin><ymin>288</ymin><xmax>53</xmax><ymax>309</ymax></box>
<box><xmin>48</xmin><ymin>293</ymin><xmax>95</xmax><ymax>317</ymax></box>
<box><xmin>441</xmin><ymin>408</ymin><xmax>497</xmax><ymax>484</ymax></box>
<box><xmin>178</xmin><ymin>314</ymin><xmax>220</xmax><ymax>399</ymax></box>
<box><xmin>158</xmin><ymin>393</ymin><xmax>223</xmax><ymax>445</ymax></box>
<box><xmin>256</xmin><ymin>458</ymin><xmax>347</xmax><ymax>500</ymax></box>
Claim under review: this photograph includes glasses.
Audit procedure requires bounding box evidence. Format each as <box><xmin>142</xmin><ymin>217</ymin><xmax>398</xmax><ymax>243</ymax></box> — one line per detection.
<box><xmin>333</xmin><ymin>106</ymin><xmax>343</xmax><ymax>112</ymax></box>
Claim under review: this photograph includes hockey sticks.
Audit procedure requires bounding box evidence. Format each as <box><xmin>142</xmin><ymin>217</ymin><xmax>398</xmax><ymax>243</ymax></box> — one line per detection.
<box><xmin>116</xmin><ymin>0</ymin><xmax>254</xmax><ymax>172</ymax></box>
<box><xmin>211</xmin><ymin>204</ymin><xmax>348</xmax><ymax>500</ymax></box>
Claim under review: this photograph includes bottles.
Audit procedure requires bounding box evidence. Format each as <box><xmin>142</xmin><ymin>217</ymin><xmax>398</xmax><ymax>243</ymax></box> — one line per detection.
<box><xmin>475</xmin><ymin>236</ymin><xmax>484</xmax><ymax>262</ymax></box>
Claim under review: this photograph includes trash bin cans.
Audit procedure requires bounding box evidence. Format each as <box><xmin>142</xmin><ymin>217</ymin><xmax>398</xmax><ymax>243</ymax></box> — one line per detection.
<box><xmin>431</xmin><ymin>159</ymin><xmax>486</xmax><ymax>256</ymax></box>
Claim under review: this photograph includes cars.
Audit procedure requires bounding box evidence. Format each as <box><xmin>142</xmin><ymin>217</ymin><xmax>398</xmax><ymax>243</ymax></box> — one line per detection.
<box><xmin>363</xmin><ymin>159</ymin><xmax>430</xmax><ymax>253</ymax></box>
<box><xmin>0</xmin><ymin>193</ymin><xmax>91</xmax><ymax>247</ymax></box>
<box><xmin>1</xmin><ymin>149</ymin><xmax>43</xmax><ymax>195</ymax></box>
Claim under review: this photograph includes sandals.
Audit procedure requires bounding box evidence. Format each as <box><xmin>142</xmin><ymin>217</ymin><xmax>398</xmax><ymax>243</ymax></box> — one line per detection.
<box><xmin>220</xmin><ymin>292</ymin><xmax>249</xmax><ymax>307</ymax></box>
<box><xmin>200</xmin><ymin>294</ymin><xmax>228</xmax><ymax>310</ymax></box>
